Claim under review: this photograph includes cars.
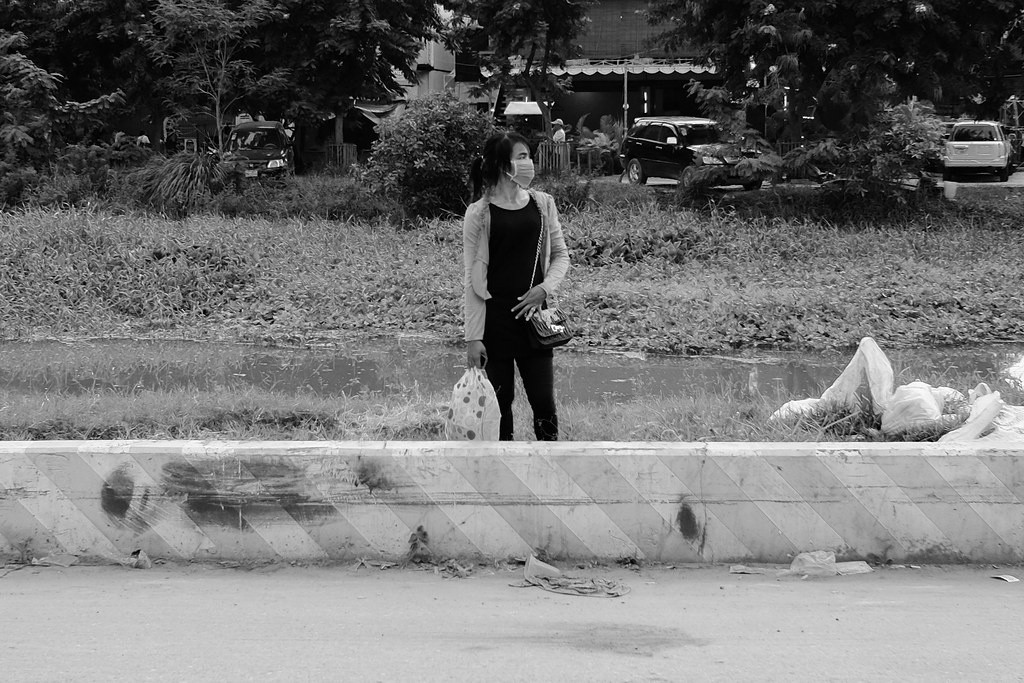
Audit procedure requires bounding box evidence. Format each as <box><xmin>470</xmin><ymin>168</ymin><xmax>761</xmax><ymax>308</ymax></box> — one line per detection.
<box><xmin>223</xmin><ymin>120</ymin><xmax>296</xmax><ymax>179</ymax></box>
<box><xmin>945</xmin><ymin>120</ymin><xmax>1017</xmax><ymax>181</ymax></box>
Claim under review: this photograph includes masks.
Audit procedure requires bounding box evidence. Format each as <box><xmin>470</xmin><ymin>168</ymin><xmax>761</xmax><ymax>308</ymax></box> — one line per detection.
<box><xmin>506</xmin><ymin>159</ymin><xmax>535</xmax><ymax>188</ymax></box>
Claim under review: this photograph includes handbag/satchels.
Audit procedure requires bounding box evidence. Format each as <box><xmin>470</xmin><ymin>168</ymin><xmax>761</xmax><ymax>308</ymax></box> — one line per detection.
<box><xmin>530</xmin><ymin>308</ymin><xmax>573</xmax><ymax>347</ymax></box>
<box><xmin>444</xmin><ymin>365</ymin><xmax>501</xmax><ymax>441</ymax></box>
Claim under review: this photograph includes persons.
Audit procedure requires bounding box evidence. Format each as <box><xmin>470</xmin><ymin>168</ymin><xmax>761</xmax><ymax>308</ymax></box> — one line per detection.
<box><xmin>115</xmin><ymin>129</ymin><xmax>166</xmax><ymax>153</ymax></box>
<box><xmin>463</xmin><ymin>132</ymin><xmax>570</xmax><ymax>441</ymax></box>
<box><xmin>214</xmin><ymin>130</ymin><xmax>224</xmax><ymax>149</ymax></box>
<box><xmin>549</xmin><ymin>119</ymin><xmax>566</xmax><ymax>168</ymax></box>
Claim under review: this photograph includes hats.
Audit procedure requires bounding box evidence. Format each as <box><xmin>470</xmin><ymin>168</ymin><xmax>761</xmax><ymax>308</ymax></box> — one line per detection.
<box><xmin>551</xmin><ymin>119</ymin><xmax>563</xmax><ymax>126</ymax></box>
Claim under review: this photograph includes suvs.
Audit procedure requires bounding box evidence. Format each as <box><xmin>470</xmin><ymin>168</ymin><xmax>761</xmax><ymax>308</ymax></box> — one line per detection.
<box><xmin>618</xmin><ymin>115</ymin><xmax>764</xmax><ymax>193</ymax></box>
